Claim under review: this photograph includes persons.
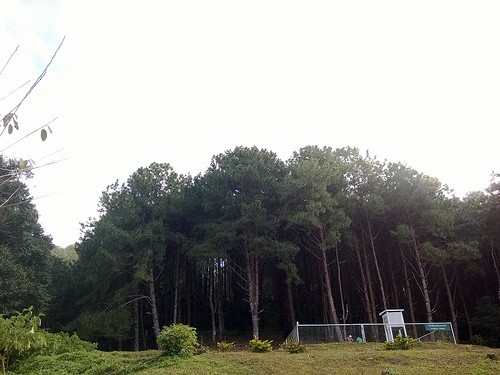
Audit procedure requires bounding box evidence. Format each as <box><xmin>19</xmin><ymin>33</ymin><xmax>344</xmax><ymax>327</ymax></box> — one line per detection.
<box><xmin>345</xmin><ymin>335</ymin><xmax>353</xmax><ymax>342</ymax></box>
<box><xmin>356</xmin><ymin>336</ymin><xmax>362</xmax><ymax>342</ymax></box>
